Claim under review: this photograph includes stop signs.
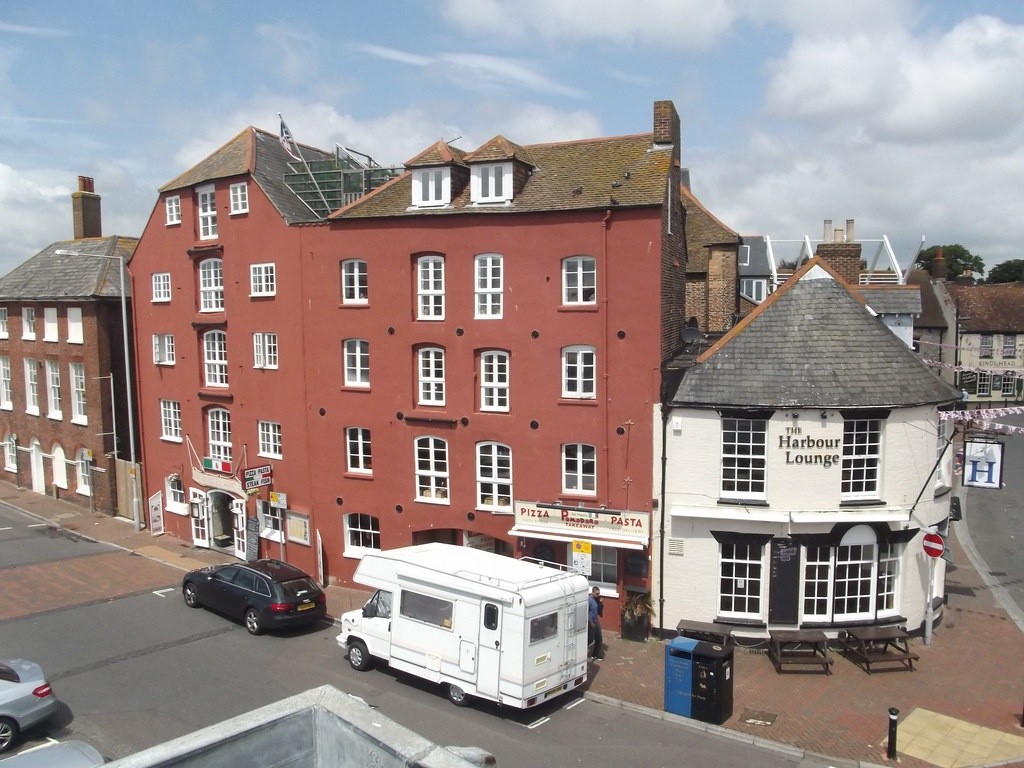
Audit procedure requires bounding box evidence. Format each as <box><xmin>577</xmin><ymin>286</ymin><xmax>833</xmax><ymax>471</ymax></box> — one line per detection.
<box><xmin>923</xmin><ymin>533</ymin><xmax>945</xmax><ymax>557</ymax></box>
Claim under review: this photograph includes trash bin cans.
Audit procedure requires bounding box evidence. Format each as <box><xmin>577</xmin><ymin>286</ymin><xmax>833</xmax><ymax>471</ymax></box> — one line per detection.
<box><xmin>664</xmin><ymin>635</ymin><xmax>734</xmax><ymax>727</ymax></box>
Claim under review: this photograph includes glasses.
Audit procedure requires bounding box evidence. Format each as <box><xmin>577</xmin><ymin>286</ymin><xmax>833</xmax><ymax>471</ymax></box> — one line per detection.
<box><xmin>595</xmin><ymin>593</ymin><xmax>599</xmax><ymax>595</ymax></box>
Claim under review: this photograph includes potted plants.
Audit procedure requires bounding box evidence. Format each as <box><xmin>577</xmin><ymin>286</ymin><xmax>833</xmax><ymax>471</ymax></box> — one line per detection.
<box><xmin>620</xmin><ymin>591</ymin><xmax>657</xmax><ymax>644</ymax></box>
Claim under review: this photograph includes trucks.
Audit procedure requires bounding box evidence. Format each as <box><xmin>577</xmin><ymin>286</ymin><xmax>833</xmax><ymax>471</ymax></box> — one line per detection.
<box><xmin>337</xmin><ymin>543</ymin><xmax>588</xmax><ymax>707</ymax></box>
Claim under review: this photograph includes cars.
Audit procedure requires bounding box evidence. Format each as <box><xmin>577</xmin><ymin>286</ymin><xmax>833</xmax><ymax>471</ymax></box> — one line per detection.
<box><xmin>183</xmin><ymin>559</ymin><xmax>330</xmax><ymax>636</ymax></box>
<box><xmin>0</xmin><ymin>659</ymin><xmax>59</xmax><ymax>753</ymax></box>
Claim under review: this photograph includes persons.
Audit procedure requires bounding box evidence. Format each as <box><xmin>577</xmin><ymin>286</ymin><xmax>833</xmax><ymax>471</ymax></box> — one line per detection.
<box><xmin>588</xmin><ymin>586</ymin><xmax>604</xmax><ymax>661</ymax></box>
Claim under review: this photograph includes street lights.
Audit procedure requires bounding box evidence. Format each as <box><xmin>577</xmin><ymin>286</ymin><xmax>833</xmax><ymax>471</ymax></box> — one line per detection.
<box><xmin>55</xmin><ymin>250</ymin><xmax>141</xmax><ymax>535</ymax></box>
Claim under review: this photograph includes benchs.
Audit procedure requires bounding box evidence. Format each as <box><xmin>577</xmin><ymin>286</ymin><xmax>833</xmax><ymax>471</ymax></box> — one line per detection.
<box><xmin>776</xmin><ymin>656</ymin><xmax>834</xmax><ymax>666</ymax></box>
<box><xmin>837</xmin><ymin>636</ymin><xmax>856</xmax><ymax>646</ymax></box>
<box><xmin>864</xmin><ymin>652</ymin><xmax>920</xmax><ymax>663</ymax></box>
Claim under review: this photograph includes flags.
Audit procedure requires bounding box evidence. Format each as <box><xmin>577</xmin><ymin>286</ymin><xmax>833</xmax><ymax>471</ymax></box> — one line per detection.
<box><xmin>279</xmin><ymin>120</ymin><xmax>303</xmax><ymax>162</ymax></box>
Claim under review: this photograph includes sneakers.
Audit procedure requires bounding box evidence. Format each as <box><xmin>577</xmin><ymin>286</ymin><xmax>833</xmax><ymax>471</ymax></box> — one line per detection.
<box><xmin>591</xmin><ymin>655</ymin><xmax>603</xmax><ymax>661</ymax></box>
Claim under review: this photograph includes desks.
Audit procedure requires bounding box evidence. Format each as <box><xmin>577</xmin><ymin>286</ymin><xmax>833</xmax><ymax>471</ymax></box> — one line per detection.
<box><xmin>843</xmin><ymin>625</ymin><xmax>914</xmax><ymax>676</ymax></box>
<box><xmin>676</xmin><ymin>619</ymin><xmax>734</xmax><ymax>645</ymax></box>
<box><xmin>768</xmin><ymin>629</ymin><xmax>831</xmax><ymax>676</ymax></box>
